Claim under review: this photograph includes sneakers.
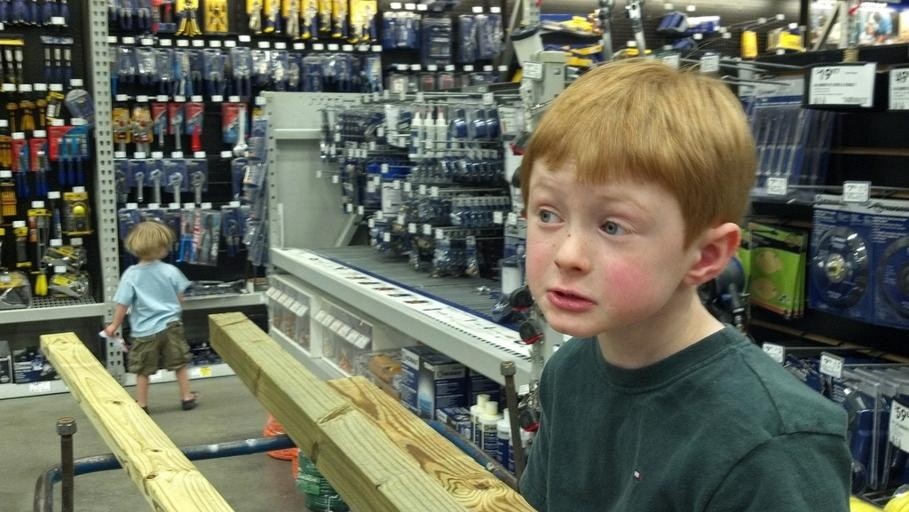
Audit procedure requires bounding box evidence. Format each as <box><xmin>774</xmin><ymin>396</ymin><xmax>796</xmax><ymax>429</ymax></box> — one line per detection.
<box><xmin>135</xmin><ymin>400</ymin><xmax>150</xmax><ymax>415</ymax></box>
<box><xmin>181</xmin><ymin>392</ymin><xmax>201</xmax><ymax>410</ymax></box>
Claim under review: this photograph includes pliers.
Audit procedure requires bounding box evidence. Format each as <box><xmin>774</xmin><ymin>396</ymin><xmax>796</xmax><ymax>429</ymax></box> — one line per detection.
<box><xmin>179</xmin><ymin>210</ymin><xmax>220</xmax><ymax>267</ymax></box>
<box><xmin>111</xmin><ymin>51</ymin><xmax>384</xmax><ymax>93</ymax></box>
<box><xmin>248</xmin><ymin>1</ymin><xmax>378</xmax><ymax>37</ymax></box>
<box><xmin>176</xmin><ymin>1</ymin><xmax>201</xmax><ymax>37</ymax></box>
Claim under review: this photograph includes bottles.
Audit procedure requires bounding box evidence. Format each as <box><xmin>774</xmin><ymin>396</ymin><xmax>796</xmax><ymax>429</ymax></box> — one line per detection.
<box><xmin>468</xmin><ymin>395</ymin><xmax>527</xmax><ymax>475</ymax></box>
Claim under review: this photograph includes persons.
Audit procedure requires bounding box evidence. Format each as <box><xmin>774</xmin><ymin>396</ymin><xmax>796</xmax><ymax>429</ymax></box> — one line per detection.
<box><xmin>512</xmin><ymin>57</ymin><xmax>849</xmax><ymax>510</ymax></box>
<box><xmin>107</xmin><ymin>219</ymin><xmax>207</xmax><ymax>413</ymax></box>
